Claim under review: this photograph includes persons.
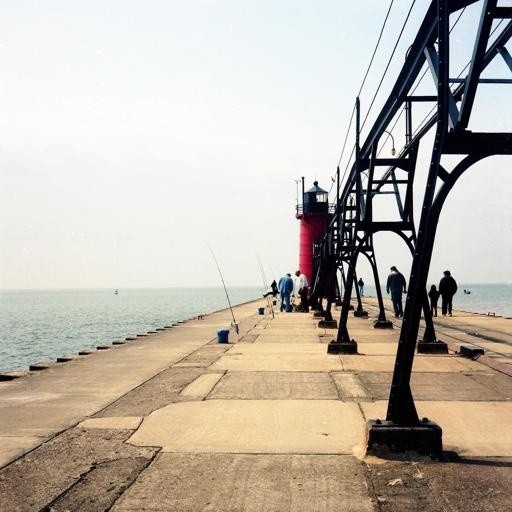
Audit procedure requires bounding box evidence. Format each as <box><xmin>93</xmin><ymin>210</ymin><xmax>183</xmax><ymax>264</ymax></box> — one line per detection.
<box><xmin>271</xmin><ymin>280</ymin><xmax>277</xmax><ymax>295</ymax></box>
<box><xmin>439</xmin><ymin>270</ymin><xmax>457</xmax><ymax>317</ymax></box>
<box><xmin>357</xmin><ymin>278</ymin><xmax>364</xmax><ymax>296</ymax></box>
<box><xmin>427</xmin><ymin>285</ymin><xmax>439</xmax><ymax>317</ymax></box>
<box><xmin>386</xmin><ymin>265</ymin><xmax>407</xmax><ymax>318</ymax></box>
<box><xmin>262</xmin><ymin>286</ymin><xmax>278</xmax><ymax>297</ymax></box>
<box><xmin>276</xmin><ymin>273</ymin><xmax>293</xmax><ymax>312</ymax></box>
<box><xmin>294</xmin><ymin>270</ymin><xmax>310</xmax><ymax>313</ymax></box>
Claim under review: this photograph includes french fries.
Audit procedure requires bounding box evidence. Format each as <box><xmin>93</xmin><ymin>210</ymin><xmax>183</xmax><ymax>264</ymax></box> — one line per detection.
<box><xmin>196</xmin><ymin>233</ymin><xmax>239</xmax><ymax>339</ymax></box>
<box><xmin>250</xmin><ymin>245</ymin><xmax>274</xmax><ymax>320</ymax></box>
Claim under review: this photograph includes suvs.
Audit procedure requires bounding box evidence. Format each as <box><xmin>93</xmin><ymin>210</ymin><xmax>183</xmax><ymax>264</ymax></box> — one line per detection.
<box><xmin>273</xmin><ymin>301</ymin><xmax>276</xmax><ymax>305</ymax></box>
<box><xmin>217</xmin><ymin>329</ymin><xmax>228</xmax><ymax>343</ymax></box>
<box><xmin>259</xmin><ymin>307</ymin><xmax>264</xmax><ymax>314</ymax></box>
